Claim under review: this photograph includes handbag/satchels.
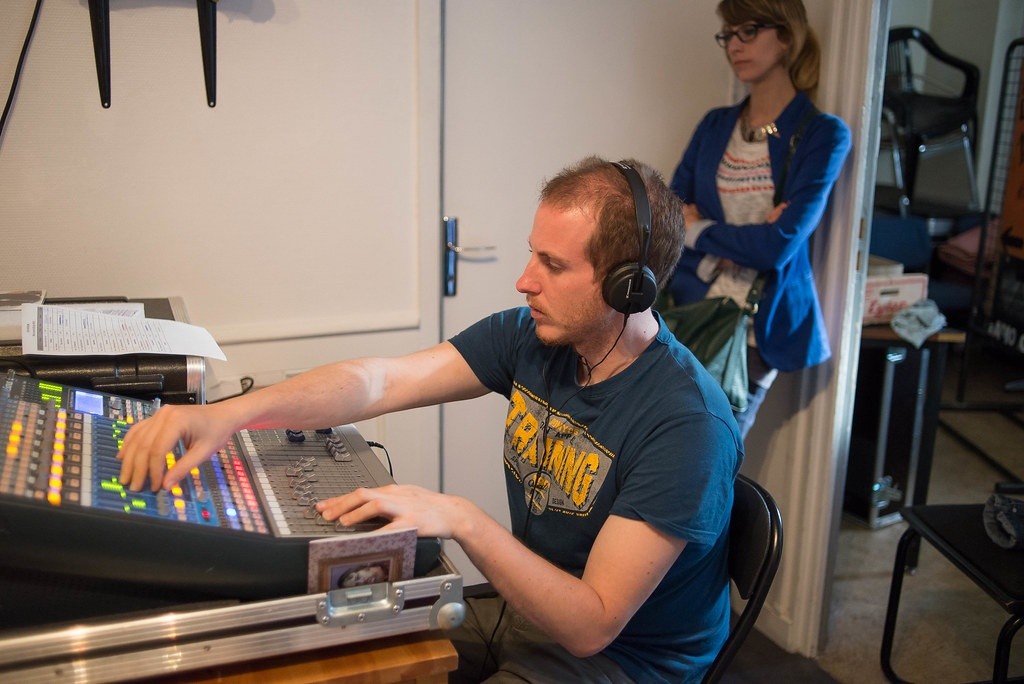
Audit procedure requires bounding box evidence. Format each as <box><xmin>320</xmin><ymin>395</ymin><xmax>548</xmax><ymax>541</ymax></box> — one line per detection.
<box><xmin>654</xmin><ymin>291</ymin><xmax>751</xmax><ymax>414</ymax></box>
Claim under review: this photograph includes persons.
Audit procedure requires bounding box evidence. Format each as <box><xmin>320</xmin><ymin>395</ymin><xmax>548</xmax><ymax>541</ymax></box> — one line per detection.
<box><xmin>672</xmin><ymin>0</ymin><xmax>854</xmax><ymax>437</ymax></box>
<box><xmin>116</xmin><ymin>156</ymin><xmax>744</xmax><ymax>683</ymax></box>
<box><xmin>338</xmin><ymin>560</ymin><xmax>389</xmax><ymax>588</ymax></box>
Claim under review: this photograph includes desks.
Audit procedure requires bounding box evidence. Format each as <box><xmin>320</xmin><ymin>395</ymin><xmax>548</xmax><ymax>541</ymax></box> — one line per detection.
<box><xmin>841</xmin><ymin>326</ymin><xmax>966</xmax><ymax>529</ymax></box>
<box><xmin>133</xmin><ymin>631</ymin><xmax>460</xmax><ymax>684</ymax></box>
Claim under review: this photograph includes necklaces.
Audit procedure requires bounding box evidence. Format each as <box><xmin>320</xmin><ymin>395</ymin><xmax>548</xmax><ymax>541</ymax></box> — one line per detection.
<box><xmin>741</xmin><ymin>104</ymin><xmax>778</xmax><ymax>143</ymax></box>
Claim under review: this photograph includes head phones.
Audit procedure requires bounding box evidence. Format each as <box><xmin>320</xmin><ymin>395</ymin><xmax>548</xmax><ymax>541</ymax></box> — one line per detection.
<box><xmin>603</xmin><ymin>159</ymin><xmax>660</xmax><ymax>315</ymax></box>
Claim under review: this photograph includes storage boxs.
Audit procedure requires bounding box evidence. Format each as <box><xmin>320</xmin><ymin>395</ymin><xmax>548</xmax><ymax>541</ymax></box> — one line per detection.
<box><xmin>864</xmin><ymin>254</ymin><xmax>929</xmax><ymax>324</ymax></box>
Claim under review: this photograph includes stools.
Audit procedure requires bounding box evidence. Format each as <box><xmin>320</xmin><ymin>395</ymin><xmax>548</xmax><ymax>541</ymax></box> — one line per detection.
<box><xmin>882</xmin><ymin>504</ymin><xmax>1024</xmax><ymax>684</ymax></box>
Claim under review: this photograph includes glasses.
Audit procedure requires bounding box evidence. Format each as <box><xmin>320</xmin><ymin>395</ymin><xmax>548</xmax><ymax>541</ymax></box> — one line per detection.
<box><xmin>714</xmin><ymin>21</ymin><xmax>782</xmax><ymax>48</ymax></box>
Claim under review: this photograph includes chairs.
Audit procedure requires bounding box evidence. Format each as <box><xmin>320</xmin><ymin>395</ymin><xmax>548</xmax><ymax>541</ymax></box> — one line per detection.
<box><xmin>880</xmin><ymin>26</ymin><xmax>983</xmax><ymax>221</ymax></box>
<box><xmin>699</xmin><ymin>473</ymin><xmax>784</xmax><ymax>684</ymax></box>
